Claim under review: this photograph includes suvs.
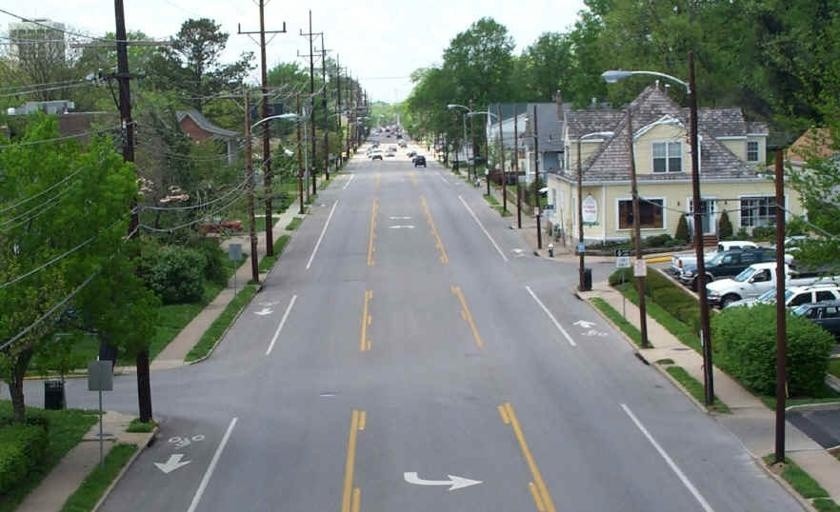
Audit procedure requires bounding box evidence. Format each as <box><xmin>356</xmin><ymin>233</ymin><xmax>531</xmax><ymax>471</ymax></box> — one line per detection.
<box><xmin>195</xmin><ymin>215</ymin><xmax>242</xmax><ymax>238</ymax></box>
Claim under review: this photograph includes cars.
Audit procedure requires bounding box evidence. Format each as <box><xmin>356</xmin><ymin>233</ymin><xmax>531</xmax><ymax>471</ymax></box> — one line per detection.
<box><xmin>670</xmin><ymin>231</ymin><xmax>838</xmax><ymax>342</ymax></box>
<box><xmin>367</xmin><ymin>125</ymin><xmax>444</xmax><ymax>168</ymax></box>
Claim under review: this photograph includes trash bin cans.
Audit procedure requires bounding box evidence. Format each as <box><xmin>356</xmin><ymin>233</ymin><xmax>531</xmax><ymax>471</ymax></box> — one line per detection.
<box><xmin>45</xmin><ymin>380</ymin><xmax>64</xmax><ymax>410</ymax></box>
<box><xmin>584</xmin><ymin>268</ymin><xmax>591</xmax><ymax>290</ymax></box>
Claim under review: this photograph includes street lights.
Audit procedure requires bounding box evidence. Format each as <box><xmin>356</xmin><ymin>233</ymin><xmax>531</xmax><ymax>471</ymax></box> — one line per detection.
<box><xmin>576</xmin><ymin>131</ymin><xmax>615</xmax><ymax>294</ymax></box>
<box><xmin>447</xmin><ymin>99</ymin><xmax>478</xmax><ymax>186</ymax></box>
<box><xmin>464</xmin><ymin>103</ymin><xmax>508</xmax><ymax>216</ymax></box>
<box><xmin>238</xmin><ymin>88</ymin><xmax>302</xmax><ymax>288</ymax></box>
<box><xmin>601</xmin><ymin>49</ymin><xmax>714</xmax><ymax>406</ymax></box>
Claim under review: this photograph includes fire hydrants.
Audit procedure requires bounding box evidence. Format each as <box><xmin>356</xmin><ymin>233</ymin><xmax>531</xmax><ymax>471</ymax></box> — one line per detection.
<box><xmin>548</xmin><ymin>242</ymin><xmax>556</xmax><ymax>257</ymax></box>
